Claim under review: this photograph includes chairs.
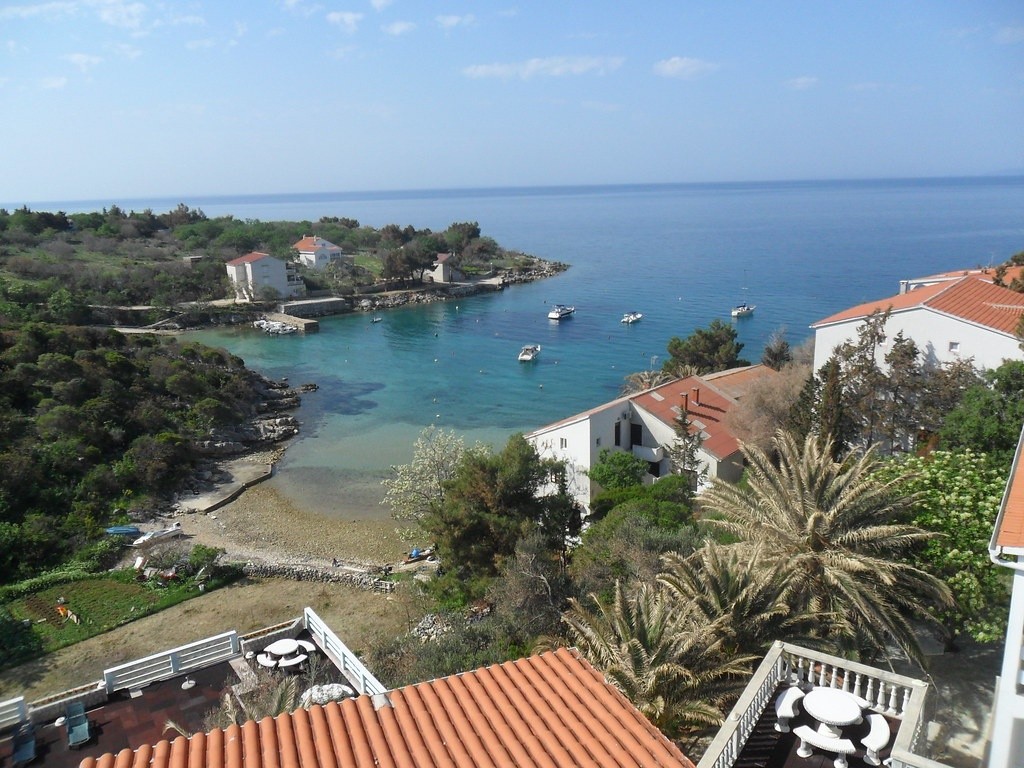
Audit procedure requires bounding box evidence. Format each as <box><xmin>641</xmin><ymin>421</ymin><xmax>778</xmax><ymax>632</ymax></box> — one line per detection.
<box><xmin>10</xmin><ymin>721</ymin><xmax>38</xmax><ymax>768</ymax></box>
<box><xmin>64</xmin><ymin>702</ymin><xmax>92</xmax><ymax>749</ymax></box>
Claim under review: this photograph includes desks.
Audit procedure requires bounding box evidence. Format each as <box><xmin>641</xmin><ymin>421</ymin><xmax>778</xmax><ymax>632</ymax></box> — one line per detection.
<box><xmin>802</xmin><ymin>688</ymin><xmax>862</xmax><ymax>741</ymax></box>
<box><xmin>263</xmin><ymin>638</ymin><xmax>305</xmax><ymax>673</ymax></box>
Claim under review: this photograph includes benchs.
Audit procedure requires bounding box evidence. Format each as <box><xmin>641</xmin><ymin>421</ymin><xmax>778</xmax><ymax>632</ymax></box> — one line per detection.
<box><xmin>811</xmin><ymin>686</ymin><xmax>871</xmax><ymax>711</ymax></box>
<box><xmin>772</xmin><ymin>686</ymin><xmax>805</xmax><ymax>734</ymax></box>
<box><xmin>792</xmin><ymin>725</ymin><xmax>857</xmax><ymax>768</ymax></box>
<box><xmin>278</xmin><ymin>655</ymin><xmax>307</xmax><ymax>671</ymax></box>
<box><xmin>256</xmin><ymin>654</ymin><xmax>278</xmax><ymax>673</ymax></box>
<box><xmin>297</xmin><ymin>640</ymin><xmax>317</xmax><ymax>653</ymax></box>
<box><xmin>859</xmin><ymin>713</ymin><xmax>891</xmax><ymax>766</ymax></box>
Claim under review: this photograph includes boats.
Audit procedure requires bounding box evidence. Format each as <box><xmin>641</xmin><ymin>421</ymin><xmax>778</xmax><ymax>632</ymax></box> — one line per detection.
<box><xmin>548</xmin><ymin>304</ymin><xmax>575</xmax><ymax>320</ymax></box>
<box><xmin>518</xmin><ymin>344</ymin><xmax>541</xmax><ymax>362</ymax></box>
<box><xmin>400</xmin><ymin>548</ymin><xmax>437</xmax><ymax>566</ymax></box>
<box><xmin>259</xmin><ymin>322</ymin><xmax>296</xmax><ymax>335</ymax></box>
<box><xmin>621</xmin><ymin>312</ymin><xmax>642</xmax><ymax>324</ymax></box>
<box><xmin>731</xmin><ymin>302</ymin><xmax>756</xmax><ymax>317</ymax></box>
<box><xmin>133</xmin><ymin>522</ymin><xmax>183</xmax><ymax>549</ymax></box>
<box><xmin>371</xmin><ymin>317</ymin><xmax>382</xmax><ymax>324</ymax></box>
